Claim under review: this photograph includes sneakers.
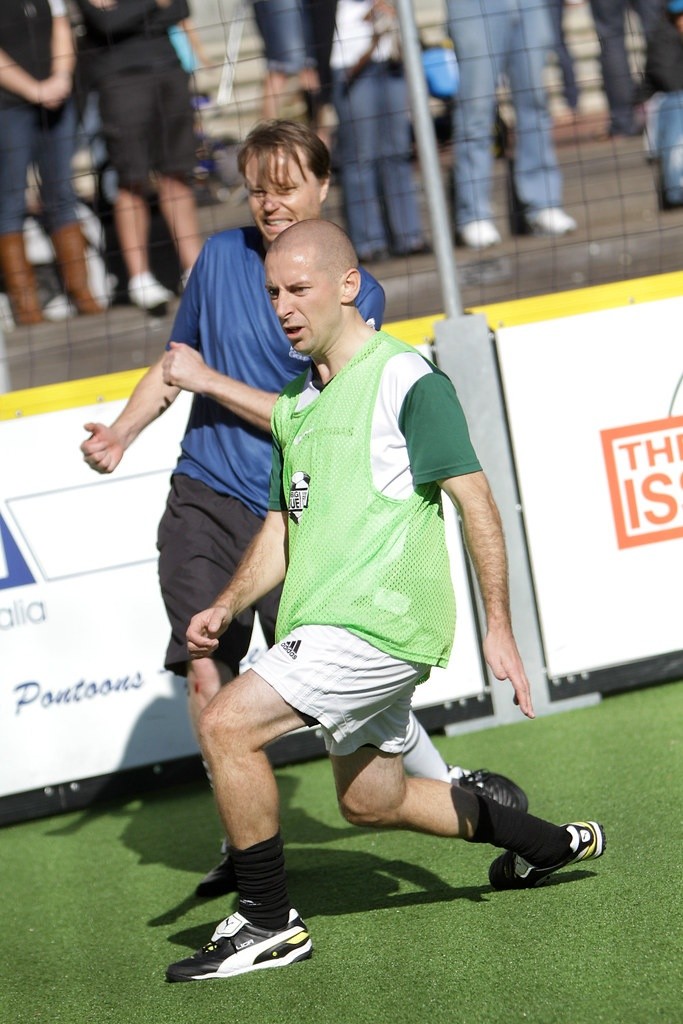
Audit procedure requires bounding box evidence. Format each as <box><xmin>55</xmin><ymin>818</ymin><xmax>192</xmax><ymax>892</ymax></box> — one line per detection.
<box><xmin>167</xmin><ymin>907</ymin><xmax>312</xmax><ymax>982</ymax></box>
<box><xmin>446</xmin><ymin>762</ymin><xmax>528</xmax><ymax>814</ymax></box>
<box><xmin>488</xmin><ymin>820</ymin><xmax>606</xmax><ymax>890</ymax></box>
<box><xmin>195</xmin><ymin>852</ymin><xmax>237</xmax><ymax>898</ymax></box>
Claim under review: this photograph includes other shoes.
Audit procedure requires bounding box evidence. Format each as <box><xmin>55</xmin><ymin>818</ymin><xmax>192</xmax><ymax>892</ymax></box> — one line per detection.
<box><xmin>523</xmin><ymin>208</ymin><xmax>577</xmax><ymax>236</ymax></box>
<box><xmin>399</xmin><ymin>242</ymin><xmax>433</xmax><ymax>257</ymax></box>
<box><xmin>459</xmin><ymin>220</ymin><xmax>500</xmax><ymax>248</ymax></box>
<box><xmin>180</xmin><ymin>268</ymin><xmax>193</xmax><ymax>288</ymax></box>
<box><xmin>127</xmin><ymin>274</ymin><xmax>174</xmax><ymax>308</ymax></box>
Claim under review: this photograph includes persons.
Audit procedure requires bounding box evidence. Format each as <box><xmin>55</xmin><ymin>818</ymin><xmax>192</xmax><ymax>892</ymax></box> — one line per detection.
<box><xmin>167</xmin><ymin>215</ymin><xmax>608</xmax><ymax>985</ymax></box>
<box><xmin>0</xmin><ymin>1</ymin><xmax>683</xmax><ymax>329</ymax></box>
<box><xmin>84</xmin><ymin>116</ymin><xmax>534</xmax><ymax>897</ymax></box>
